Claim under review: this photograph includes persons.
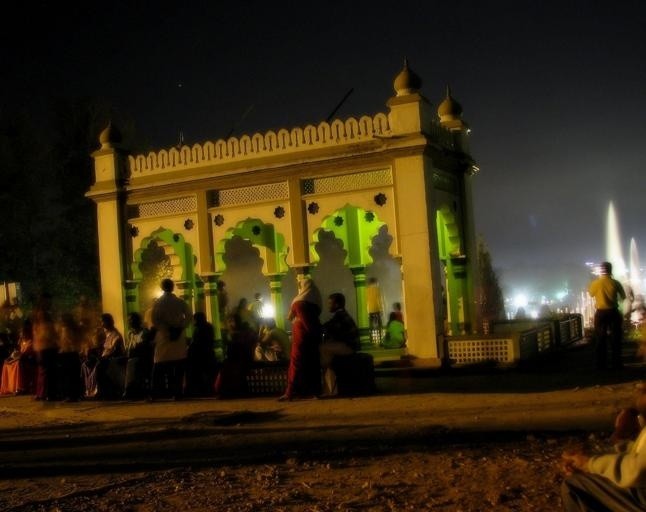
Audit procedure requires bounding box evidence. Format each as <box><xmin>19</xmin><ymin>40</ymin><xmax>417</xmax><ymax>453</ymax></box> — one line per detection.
<box><xmin>441</xmin><ymin>285</ymin><xmax>449</xmax><ymax>334</ymax></box>
<box><xmin>562</xmin><ymin>378</ymin><xmax>646</xmax><ymax>511</ymax></box>
<box><xmin>385</xmin><ymin>303</ymin><xmax>407</xmax><ymax>350</ymax></box>
<box><xmin>366</xmin><ymin>276</ymin><xmax>385</xmax><ymax>347</ymax></box>
<box><xmin>588</xmin><ymin>261</ymin><xmax>626</xmax><ymax>385</ymax></box>
